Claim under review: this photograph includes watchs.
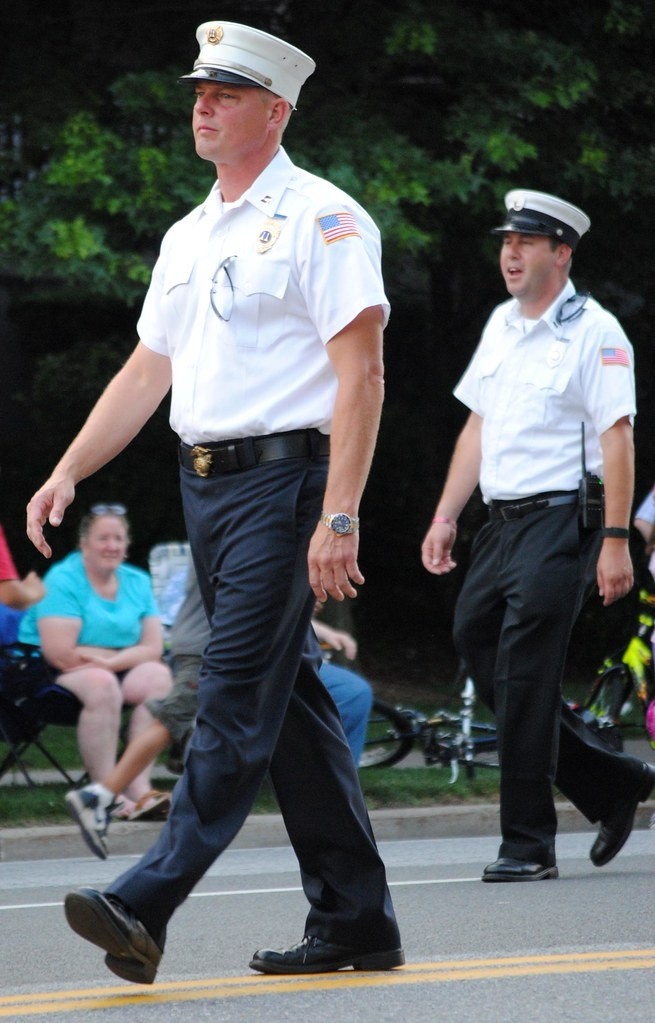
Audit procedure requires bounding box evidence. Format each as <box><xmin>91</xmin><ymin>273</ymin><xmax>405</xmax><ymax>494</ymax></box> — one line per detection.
<box><xmin>321</xmin><ymin>512</ymin><xmax>359</xmax><ymax>538</ymax></box>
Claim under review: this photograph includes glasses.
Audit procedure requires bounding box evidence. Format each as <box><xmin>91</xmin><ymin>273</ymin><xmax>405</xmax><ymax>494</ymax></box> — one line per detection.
<box><xmin>91</xmin><ymin>504</ymin><xmax>127</xmax><ymax>518</ymax></box>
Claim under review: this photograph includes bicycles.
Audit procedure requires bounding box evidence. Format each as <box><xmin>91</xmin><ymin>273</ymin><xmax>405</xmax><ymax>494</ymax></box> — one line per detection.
<box><xmin>360</xmin><ymin>675</ymin><xmax>621</xmax><ymax>786</ymax></box>
<box><xmin>583</xmin><ymin>586</ymin><xmax>654</xmax><ymax>715</ymax></box>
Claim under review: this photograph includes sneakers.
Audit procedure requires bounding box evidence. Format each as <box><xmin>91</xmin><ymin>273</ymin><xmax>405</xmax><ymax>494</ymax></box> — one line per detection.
<box><xmin>127</xmin><ymin>792</ymin><xmax>171</xmax><ymax>819</ymax></box>
<box><xmin>110</xmin><ymin>794</ymin><xmax>132</xmax><ymax>818</ymax></box>
<box><xmin>67</xmin><ymin>785</ymin><xmax>110</xmax><ymax>861</ymax></box>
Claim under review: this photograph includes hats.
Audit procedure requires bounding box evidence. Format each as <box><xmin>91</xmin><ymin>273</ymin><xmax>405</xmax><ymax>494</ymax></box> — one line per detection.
<box><xmin>494</xmin><ymin>188</ymin><xmax>590</xmax><ymax>251</ymax></box>
<box><xmin>180</xmin><ymin>22</ymin><xmax>314</xmax><ymax>108</ymax></box>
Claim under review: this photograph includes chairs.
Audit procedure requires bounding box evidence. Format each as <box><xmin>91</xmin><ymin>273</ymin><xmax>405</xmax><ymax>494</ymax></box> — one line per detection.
<box><xmin>0</xmin><ymin>640</ymin><xmax>135</xmax><ymax>792</ymax></box>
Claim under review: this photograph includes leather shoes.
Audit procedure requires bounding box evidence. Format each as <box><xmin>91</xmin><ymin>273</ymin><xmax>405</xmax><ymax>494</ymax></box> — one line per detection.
<box><xmin>591</xmin><ymin>762</ymin><xmax>655</xmax><ymax>868</ymax></box>
<box><xmin>249</xmin><ymin>932</ymin><xmax>404</xmax><ymax>971</ymax></box>
<box><xmin>481</xmin><ymin>856</ymin><xmax>559</xmax><ymax>882</ymax></box>
<box><xmin>64</xmin><ymin>886</ymin><xmax>161</xmax><ymax>985</ymax></box>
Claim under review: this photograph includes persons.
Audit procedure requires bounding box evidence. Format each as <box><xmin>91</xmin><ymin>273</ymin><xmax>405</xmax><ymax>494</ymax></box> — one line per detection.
<box><xmin>0</xmin><ymin>525</ymin><xmax>47</xmax><ymax>611</ymax></box>
<box><xmin>309</xmin><ymin>618</ymin><xmax>373</xmax><ymax>768</ymax></box>
<box><xmin>421</xmin><ymin>189</ymin><xmax>655</xmax><ymax>882</ymax></box>
<box><xmin>633</xmin><ymin>487</ymin><xmax>655</xmax><ymax>749</ymax></box>
<box><xmin>10</xmin><ymin>504</ymin><xmax>173</xmax><ymax>822</ymax></box>
<box><xmin>26</xmin><ymin>20</ymin><xmax>406</xmax><ymax>984</ymax></box>
<box><xmin>63</xmin><ymin>566</ymin><xmax>211</xmax><ymax>860</ymax></box>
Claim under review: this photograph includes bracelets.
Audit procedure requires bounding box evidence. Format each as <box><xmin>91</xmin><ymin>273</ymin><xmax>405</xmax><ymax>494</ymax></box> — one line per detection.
<box><xmin>603</xmin><ymin>528</ymin><xmax>630</xmax><ymax>538</ymax></box>
<box><xmin>432</xmin><ymin>518</ymin><xmax>456</xmax><ymax>529</ymax></box>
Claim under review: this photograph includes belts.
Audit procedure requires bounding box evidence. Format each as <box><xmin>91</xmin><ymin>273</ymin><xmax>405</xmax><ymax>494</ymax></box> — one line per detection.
<box><xmin>178</xmin><ymin>429</ymin><xmax>331</xmax><ymax>478</ymax></box>
<box><xmin>489</xmin><ymin>494</ymin><xmax>580</xmax><ymax>522</ymax></box>
